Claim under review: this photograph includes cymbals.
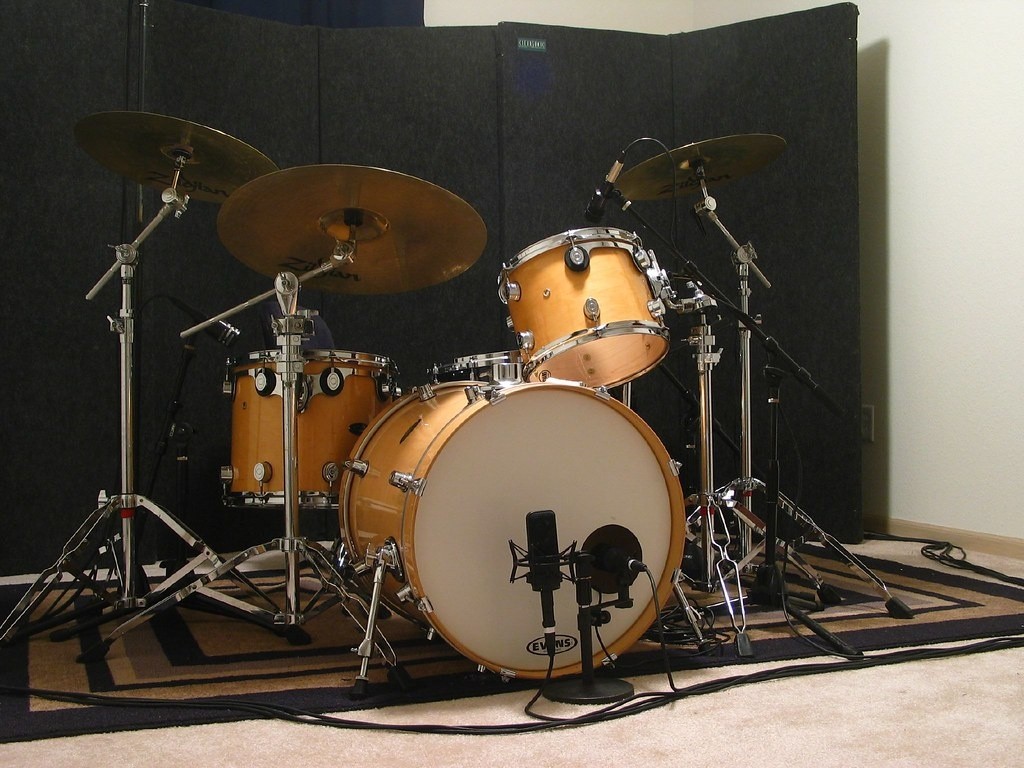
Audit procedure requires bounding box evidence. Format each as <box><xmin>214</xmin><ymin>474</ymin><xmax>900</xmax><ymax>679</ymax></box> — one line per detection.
<box><xmin>614</xmin><ymin>132</ymin><xmax>788</xmax><ymax>201</ymax></box>
<box><xmin>74</xmin><ymin>110</ymin><xmax>281</xmax><ymax>204</ymax></box>
<box><xmin>216</xmin><ymin>163</ymin><xmax>489</xmax><ymax>294</ymax></box>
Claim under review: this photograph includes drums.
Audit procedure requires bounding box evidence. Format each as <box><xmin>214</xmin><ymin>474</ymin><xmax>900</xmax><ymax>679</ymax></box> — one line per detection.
<box><xmin>338</xmin><ymin>380</ymin><xmax>687</xmax><ymax>680</ymax></box>
<box><xmin>223</xmin><ymin>349</ymin><xmax>394</xmax><ymax>511</ymax></box>
<box><xmin>426</xmin><ymin>349</ymin><xmax>524</xmax><ymax>382</ymax></box>
<box><xmin>501</xmin><ymin>227</ymin><xmax>670</xmax><ymax>391</ymax></box>
